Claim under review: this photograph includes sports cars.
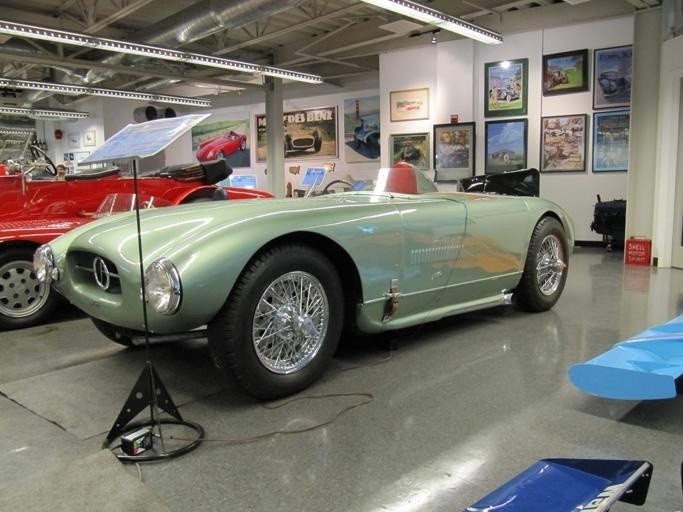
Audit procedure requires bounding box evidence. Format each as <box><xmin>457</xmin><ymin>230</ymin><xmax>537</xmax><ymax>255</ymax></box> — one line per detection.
<box><xmin>196</xmin><ymin>131</ymin><xmax>246</xmax><ymax>162</ymax></box>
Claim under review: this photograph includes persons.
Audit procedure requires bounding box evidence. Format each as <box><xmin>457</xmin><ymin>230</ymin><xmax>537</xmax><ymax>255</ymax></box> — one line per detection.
<box><xmin>55</xmin><ymin>164</ymin><xmax>67</xmax><ymax>179</ymax></box>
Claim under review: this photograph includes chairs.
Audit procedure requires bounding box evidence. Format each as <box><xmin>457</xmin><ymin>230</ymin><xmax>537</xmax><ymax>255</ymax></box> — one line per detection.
<box><xmin>383</xmin><ymin>161</ymin><xmax>417</xmax><ymax>195</ymax></box>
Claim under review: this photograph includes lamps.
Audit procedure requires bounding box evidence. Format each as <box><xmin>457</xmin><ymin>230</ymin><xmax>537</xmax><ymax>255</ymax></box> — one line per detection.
<box><xmin>1</xmin><ymin>105</ymin><xmax>91</xmax><ymax>119</ymax></box>
<box><xmin>357</xmin><ymin>0</ymin><xmax>505</xmax><ymax>47</ymax></box>
<box><xmin>1</xmin><ymin>75</ymin><xmax>213</xmax><ymax>109</ymax></box>
<box><xmin>0</xmin><ymin>138</ymin><xmax>34</xmax><ymax>146</ymax></box>
<box><xmin>1</xmin><ymin>19</ymin><xmax>323</xmax><ymax>84</ymax></box>
<box><xmin>1</xmin><ymin>127</ymin><xmax>36</xmax><ymax>135</ymax></box>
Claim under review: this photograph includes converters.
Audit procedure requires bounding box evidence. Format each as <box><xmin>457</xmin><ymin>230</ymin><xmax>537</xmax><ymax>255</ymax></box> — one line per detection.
<box><xmin>120</xmin><ymin>427</ymin><xmax>155</xmax><ymax>455</ymax></box>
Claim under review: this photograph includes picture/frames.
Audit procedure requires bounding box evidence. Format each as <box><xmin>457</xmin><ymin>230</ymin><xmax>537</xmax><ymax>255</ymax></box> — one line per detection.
<box><xmin>252</xmin><ymin>105</ymin><xmax>339</xmax><ymax>164</ymax></box>
<box><xmin>592</xmin><ymin>109</ymin><xmax>630</xmax><ymax>172</ymax></box>
<box><xmin>485</xmin><ymin>118</ymin><xmax>528</xmax><ymax>175</ymax></box>
<box><xmin>433</xmin><ymin>121</ymin><xmax>476</xmax><ymax>183</ymax></box>
<box><xmin>540</xmin><ymin>114</ymin><xmax>587</xmax><ymax>173</ymax></box>
<box><xmin>541</xmin><ymin>48</ymin><xmax>589</xmax><ymax>97</ymax></box>
<box><xmin>484</xmin><ymin>57</ymin><xmax>529</xmax><ymax>117</ymax></box>
<box><xmin>390</xmin><ymin>87</ymin><xmax>430</xmax><ymax>122</ymax></box>
<box><xmin>388</xmin><ymin>131</ymin><xmax>431</xmax><ymax>170</ymax></box>
<box><xmin>592</xmin><ymin>44</ymin><xmax>632</xmax><ymax>110</ymax></box>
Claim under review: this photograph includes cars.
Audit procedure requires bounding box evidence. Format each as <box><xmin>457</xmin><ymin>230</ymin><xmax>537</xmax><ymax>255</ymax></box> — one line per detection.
<box><xmin>490</xmin><ymin>85</ymin><xmax>519</xmax><ymax>101</ymax></box>
<box><xmin>492</xmin><ymin>149</ymin><xmax>517</xmax><ymax>162</ymax></box>
<box><xmin>285</xmin><ymin>126</ymin><xmax>323</xmax><ymax>155</ymax></box>
<box><xmin>599</xmin><ymin>70</ymin><xmax>632</xmax><ymax>98</ymax></box>
<box><xmin>354</xmin><ymin>113</ymin><xmax>380</xmax><ymax>159</ymax></box>
